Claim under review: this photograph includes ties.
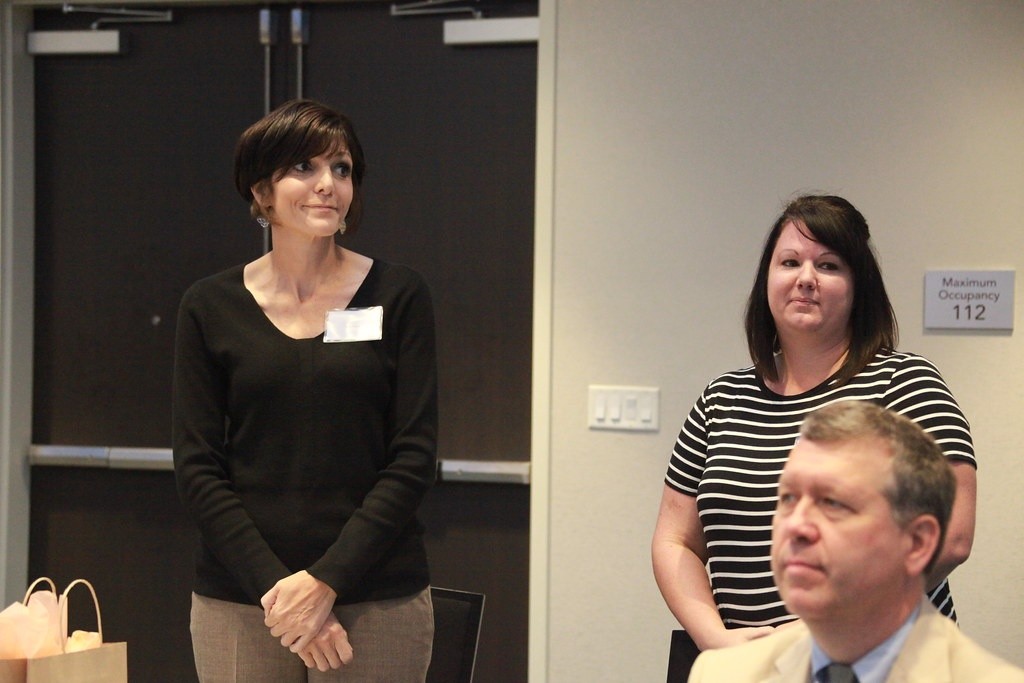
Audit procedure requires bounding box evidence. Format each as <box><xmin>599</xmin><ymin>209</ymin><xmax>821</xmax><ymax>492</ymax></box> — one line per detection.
<box><xmin>827</xmin><ymin>666</ymin><xmax>853</xmax><ymax>683</ymax></box>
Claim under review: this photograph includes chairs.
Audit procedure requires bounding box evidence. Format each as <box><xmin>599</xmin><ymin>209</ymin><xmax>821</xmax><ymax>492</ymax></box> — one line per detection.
<box><xmin>666</xmin><ymin>630</ymin><xmax>701</xmax><ymax>683</ymax></box>
<box><xmin>423</xmin><ymin>585</ymin><xmax>485</xmax><ymax>683</ymax></box>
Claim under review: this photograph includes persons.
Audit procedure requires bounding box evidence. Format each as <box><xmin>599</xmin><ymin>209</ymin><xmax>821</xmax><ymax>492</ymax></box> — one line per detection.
<box><xmin>169</xmin><ymin>100</ymin><xmax>436</xmax><ymax>683</ymax></box>
<box><xmin>688</xmin><ymin>400</ymin><xmax>1024</xmax><ymax>683</ymax></box>
<box><xmin>651</xmin><ymin>194</ymin><xmax>978</xmax><ymax>651</ymax></box>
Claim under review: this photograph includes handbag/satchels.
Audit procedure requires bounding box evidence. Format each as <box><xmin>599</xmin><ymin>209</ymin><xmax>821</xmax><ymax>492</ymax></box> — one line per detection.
<box><xmin>0</xmin><ymin>577</ymin><xmax>128</xmax><ymax>683</ymax></box>
